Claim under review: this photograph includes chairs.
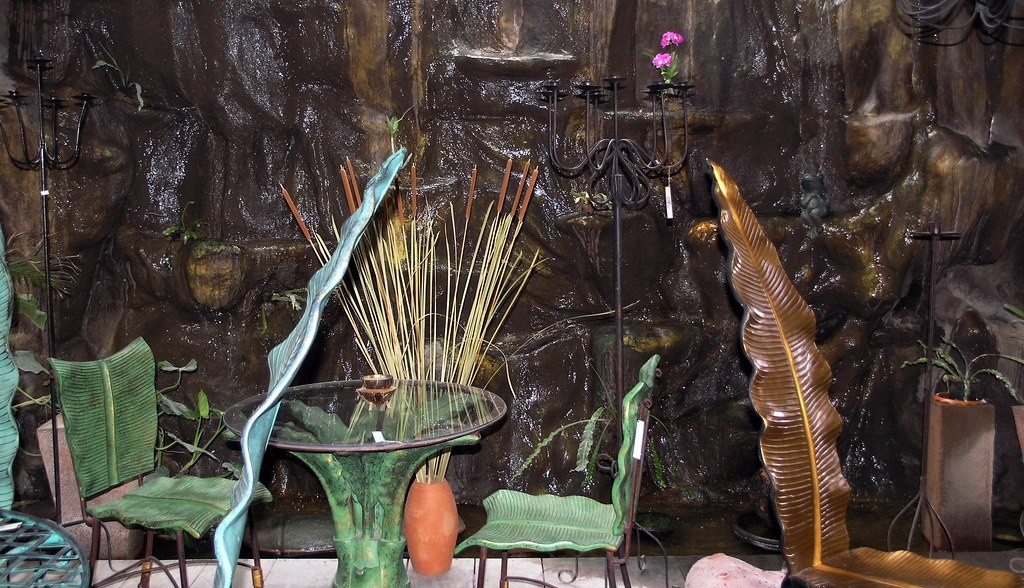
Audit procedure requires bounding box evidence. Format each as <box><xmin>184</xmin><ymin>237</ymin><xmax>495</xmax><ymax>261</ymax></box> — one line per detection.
<box><xmin>455</xmin><ymin>354</ymin><xmax>661</xmax><ymax>588</ymax></box>
<box><xmin>47</xmin><ymin>336</ymin><xmax>275</xmax><ymax>588</ymax></box>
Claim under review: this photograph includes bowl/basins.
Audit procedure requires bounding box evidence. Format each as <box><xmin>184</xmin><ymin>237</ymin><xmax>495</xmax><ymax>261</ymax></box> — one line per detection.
<box><xmin>361</xmin><ymin>374</ymin><xmax>393</xmax><ymax>389</ymax></box>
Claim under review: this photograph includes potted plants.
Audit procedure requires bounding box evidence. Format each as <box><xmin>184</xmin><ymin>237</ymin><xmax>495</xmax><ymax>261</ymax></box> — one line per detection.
<box><xmin>275</xmin><ymin>146</ymin><xmax>542</xmax><ymax>577</ymax></box>
<box><xmin>901</xmin><ymin>334</ymin><xmax>1024</xmax><ymax>405</ymax></box>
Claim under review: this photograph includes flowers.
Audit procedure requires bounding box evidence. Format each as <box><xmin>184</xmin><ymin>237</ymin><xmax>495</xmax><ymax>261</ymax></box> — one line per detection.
<box><xmin>651</xmin><ymin>30</ymin><xmax>684</xmax><ymax>83</ymax></box>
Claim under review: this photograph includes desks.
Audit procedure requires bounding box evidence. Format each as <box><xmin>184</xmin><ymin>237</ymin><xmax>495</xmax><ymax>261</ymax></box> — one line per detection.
<box><xmin>222</xmin><ymin>375</ymin><xmax>507</xmax><ymax>588</ymax></box>
